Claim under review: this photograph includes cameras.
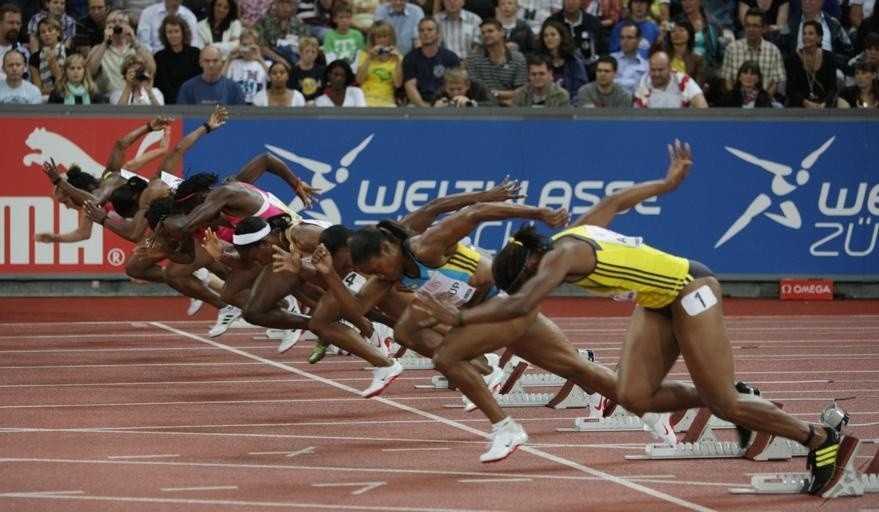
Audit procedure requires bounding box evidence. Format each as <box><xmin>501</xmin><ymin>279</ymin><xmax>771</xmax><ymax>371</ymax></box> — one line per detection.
<box><xmin>446</xmin><ymin>98</ymin><xmax>477</xmax><ymax>108</ymax></box>
<box><xmin>663</xmin><ymin>21</ymin><xmax>676</xmax><ymax>31</ymax></box>
<box><xmin>112</xmin><ymin>24</ymin><xmax>124</xmax><ymax>35</ymax></box>
<box><xmin>379</xmin><ymin>47</ymin><xmax>391</xmax><ymax>55</ymax></box>
<box><xmin>134</xmin><ymin>65</ymin><xmax>150</xmax><ymax>82</ymax></box>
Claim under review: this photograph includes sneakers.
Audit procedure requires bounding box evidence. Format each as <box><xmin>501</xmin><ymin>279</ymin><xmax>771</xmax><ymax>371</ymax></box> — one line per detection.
<box><xmin>284</xmin><ymin>293</ymin><xmax>302</xmax><ymax>315</ymax></box>
<box><xmin>278</xmin><ymin>327</ymin><xmax>304</xmax><ymax>353</ymax></box>
<box><xmin>585</xmin><ymin>392</ymin><xmax>610</xmax><ymax>417</ymax></box>
<box><xmin>484</xmin><ymin>353</ymin><xmax>501</xmax><ymax>366</ymax></box>
<box><xmin>308</xmin><ymin>337</ymin><xmax>325</xmax><ymax>363</ymax></box>
<box><xmin>209</xmin><ymin>305</ymin><xmax>242</xmax><ymax>338</ymax></box>
<box><xmin>734</xmin><ymin>381</ymin><xmax>761</xmax><ymax>452</ymax></box>
<box><xmin>361</xmin><ymin>358</ymin><xmax>403</xmax><ymax>398</ymax></box>
<box><xmin>808</xmin><ymin>427</ymin><xmax>840</xmax><ymax>493</ymax></box>
<box><xmin>465</xmin><ymin>365</ymin><xmax>507</xmax><ymax>412</ymax></box>
<box><xmin>578</xmin><ymin>348</ymin><xmax>595</xmax><ymax>363</ymax></box>
<box><xmin>187</xmin><ymin>276</ymin><xmax>210</xmax><ymax>316</ymax></box>
<box><xmin>650</xmin><ymin>413</ymin><xmax>678</xmax><ymax>444</ymax></box>
<box><xmin>480</xmin><ymin>424</ymin><xmax>529</xmax><ymax>463</ymax></box>
<box><xmin>367</xmin><ymin>321</ymin><xmax>394</xmax><ymax>358</ymax></box>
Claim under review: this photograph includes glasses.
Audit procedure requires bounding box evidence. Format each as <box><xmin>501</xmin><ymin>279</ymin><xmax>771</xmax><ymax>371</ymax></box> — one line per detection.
<box><xmin>620</xmin><ymin>35</ymin><xmax>635</xmax><ymax>39</ymax></box>
<box><xmin>596</xmin><ymin>69</ymin><xmax>612</xmax><ymax>72</ymax></box>
<box><xmin>743</xmin><ymin>23</ymin><xmax>764</xmax><ymax>28</ymax></box>
<box><xmin>530</xmin><ymin>71</ymin><xmax>545</xmax><ymax>76</ymax></box>
<box><xmin>544</xmin><ymin>32</ymin><xmax>555</xmax><ymax>37</ymax></box>
<box><xmin>481</xmin><ymin>29</ymin><xmax>495</xmax><ymax>34</ymax></box>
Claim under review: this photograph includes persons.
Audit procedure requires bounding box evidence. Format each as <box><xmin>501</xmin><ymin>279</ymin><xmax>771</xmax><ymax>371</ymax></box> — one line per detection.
<box><xmin>405</xmin><ymin>137</ymin><xmax>840</xmax><ymax>497</ymax></box>
<box><xmin>21</xmin><ymin>102</ymin><xmax>681</xmax><ymax>465</ymax></box>
<box><xmin>1</xmin><ymin>1</ymin><xmax>879</xmax><ymax>112</ymax></box>
